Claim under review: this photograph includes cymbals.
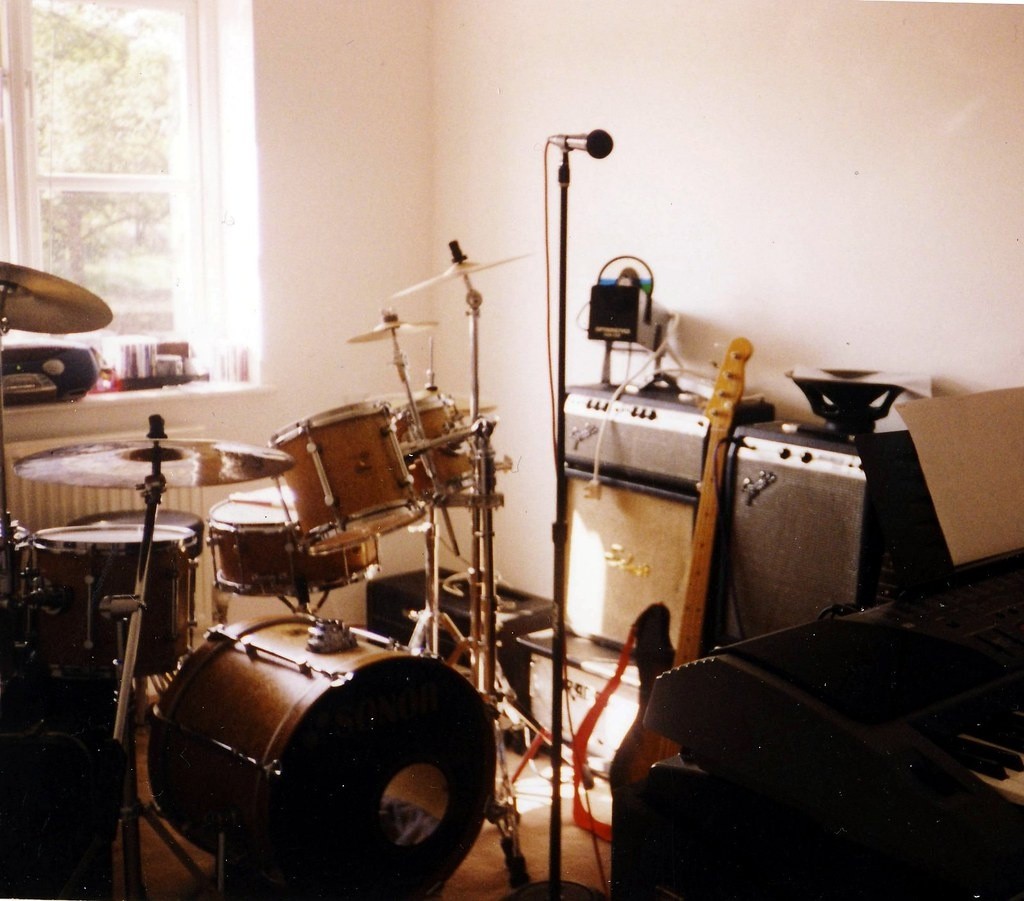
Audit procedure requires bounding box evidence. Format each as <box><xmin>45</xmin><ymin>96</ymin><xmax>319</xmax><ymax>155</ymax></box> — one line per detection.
<box><xmin>345</xmin><ymin>318</ymin><xmax>440</xmax><ymax>344</ymax></box>
<box><xmin>0</xmin><ymin>260</ymin><xmax>114</xmax><ymax>336</ymax></box>
<box><xmin>12</xmin><ymin>437</ymin><xmax>296</xmax><ymax>490</ymax></box>
<box><xmin>386</xmin><ymin>251</ymin><xmax>524</xmax><ymax>303</ymax></box>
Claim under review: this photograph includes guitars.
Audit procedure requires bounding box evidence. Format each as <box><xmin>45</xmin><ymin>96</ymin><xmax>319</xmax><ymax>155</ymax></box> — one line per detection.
<box><xmin>609</xmin><ymin>336</ymin><xmax>754</xmax><ymax>804</ymax></box>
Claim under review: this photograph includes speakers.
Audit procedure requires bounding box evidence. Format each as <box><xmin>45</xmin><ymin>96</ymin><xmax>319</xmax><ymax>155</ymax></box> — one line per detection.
<box><xmin>702</xmin><ymin>409</ymin><xmax>914</xmax><ymax>647</ymax></box>
<box><xmin>560</xmin><ymin>380</ymin><xmax>778</xmax><ymax>494</ymax></box>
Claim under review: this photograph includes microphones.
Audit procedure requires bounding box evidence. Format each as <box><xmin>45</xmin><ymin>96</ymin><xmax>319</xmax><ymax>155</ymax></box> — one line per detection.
<box><xmin>546</xmin><ymin>130</ymin><xmax>614</xmax><ymax>159</ymax></box>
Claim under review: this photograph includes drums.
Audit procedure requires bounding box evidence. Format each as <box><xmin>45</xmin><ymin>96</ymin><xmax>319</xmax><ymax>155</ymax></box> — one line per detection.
<box><xmin>267</xmin><ymin>399</ymin><xmax>417</xmax><ymax>538</ymax></box>
<box><xmin>31</xmin><ymin>523</ymin><xmax>200</xmax><ymax>677</ymax></box>
<box><xmin>207</xmin><ymin>484</ymin><xmax>326</xmax><ymax>597</ymax></box>
<box><xmin>309</xmin><ymin>529</ymin><xmax>382</xmax><ymax>592</ymax></box>
<box><xmin>145</xmin><ymin>609</ymin><xmax>498</xmax><ymax>901</ymax></box>
<box><xmin>388</xmin><ymin>392</ymin><xmax>477</xmax><ymax>501</ymax></box>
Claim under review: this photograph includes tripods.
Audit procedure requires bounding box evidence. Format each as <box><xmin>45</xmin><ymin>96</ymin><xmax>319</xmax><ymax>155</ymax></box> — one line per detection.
<box><xmin>377</xmin><ymin>443</ymin><xmax>594</xmax><ymax>882</ymax></box>
